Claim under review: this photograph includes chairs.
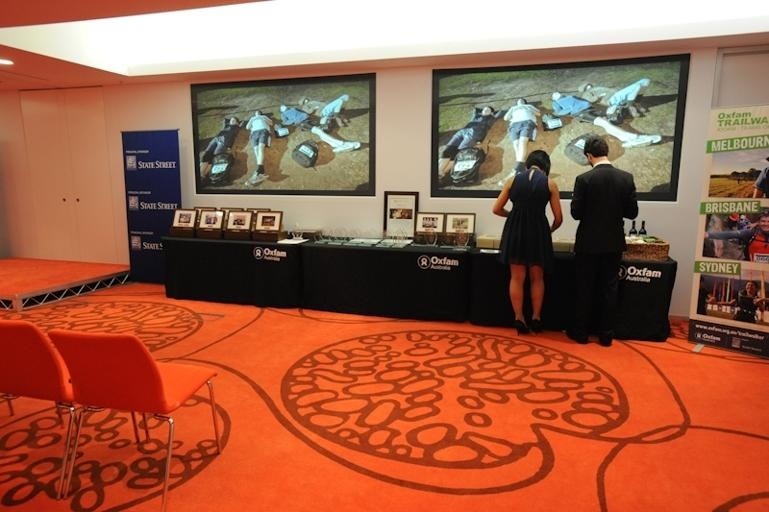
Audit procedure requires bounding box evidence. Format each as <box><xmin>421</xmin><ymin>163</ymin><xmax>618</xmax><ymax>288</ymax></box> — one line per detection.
<box><xmin>48</xmin><ymin>329</ymin><xmax>221</xmax><ymax>512</ymax></box>
<box><xmin>0</xmin><ymin>320</ymin><xmax>84</xmax><ymax>500</ymax></box>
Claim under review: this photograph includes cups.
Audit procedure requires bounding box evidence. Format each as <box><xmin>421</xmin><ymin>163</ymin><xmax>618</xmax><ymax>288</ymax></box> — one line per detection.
<box><xmin>292</xmin><ymin>224</ymin><xmax>303</xmax><ymax>240</ymax></box>
<box><xmin>424</xmin><ymin>229</ymin><xmax>438</xmax><ymax>246</ymax></box>
<box><xmin>455</xmin><ymin>230</ymin><xmax>469</xmax><ymax>248</ymax></box>
<box><xmin>382</xmin><ymin>232</ymin><xmax>394</xmax><ymax>245</ymax></box>
<box><xmin>314</xmin><ymin>224</ymin><xmax>362</xmax><ymax>244</ymax></box>
<box><xmin>397</xmin><ymin>232</ymin><xmax>407</xmax><ymax>245</ymax></box>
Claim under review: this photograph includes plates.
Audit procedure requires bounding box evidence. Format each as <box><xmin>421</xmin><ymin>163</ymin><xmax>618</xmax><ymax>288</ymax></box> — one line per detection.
<box><xmin>352</xmin><ymin>238</ymin><xmax>381</xmax><ymax>245</ymax></box>
<box><xmin>277</xmin><ymin>239</ymin><xmax>310</xmax><ymax>244</ymax></box>
<box><xmin>383</xmin><ymin>238</ymin><xmax>413</xmax><ymax>245</ymax></box>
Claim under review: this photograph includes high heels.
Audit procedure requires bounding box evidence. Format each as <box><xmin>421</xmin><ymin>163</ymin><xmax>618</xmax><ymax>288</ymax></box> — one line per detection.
<box><xmin>514</xmin><ymin>320</ymin><xmax>529</xmax><ymax>336</ymax></box>
<box><xmin>531</xmin><ymin>319</ymin><xmax>543</xmax><ymax>335</ymax></box>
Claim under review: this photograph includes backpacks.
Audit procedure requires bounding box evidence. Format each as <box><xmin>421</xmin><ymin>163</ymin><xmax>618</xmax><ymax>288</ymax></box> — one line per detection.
<box><xmin>292</xmin><ymin>139</ymin><xmax>318</xmax><ymax>168</ymax></box>
<box><xmin>451</xmin><ymin>147</ymin><xmax>484</xmax><ymax>186</ymax></box>
<box><xmin>565</xmin><ymin>133</ymin><xmax>597</xmax><ymax>165</ymax></box>
<box><xmin>209</xmin><ymin>153</ymin><xmax>234</xmax><ymax>185</ymax></box>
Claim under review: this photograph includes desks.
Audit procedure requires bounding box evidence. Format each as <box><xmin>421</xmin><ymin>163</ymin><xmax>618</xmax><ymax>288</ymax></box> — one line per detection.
<box><xmin>159</xmin><ymin>236</ymin><xmax>678</xmax><ymax>342</ymax></box>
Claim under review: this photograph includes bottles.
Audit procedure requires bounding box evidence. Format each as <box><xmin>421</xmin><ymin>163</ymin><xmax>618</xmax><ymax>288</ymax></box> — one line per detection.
<box><xmin>629</xmin><ymin>221</ymin><xmax>637</xmax><ymax>237</ymax></box>
<box><xmin>639</xmin><ymin>221</ymin><xmax>647</xmax><ymax>237</ymax></box>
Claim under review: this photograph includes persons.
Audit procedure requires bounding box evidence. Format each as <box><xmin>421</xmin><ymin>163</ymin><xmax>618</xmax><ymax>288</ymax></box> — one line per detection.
<box><xmin>199</xmin><ymin>115</ymin><xmax>245</xmax><ymax>189</ymax></box>
<box><xmin>751</xmin><ymin>156</ymin><xmax>769</xmax><ymax>198</ymax></box>
<box><xmin>498</xmin><ymin>98</ymin><xmax>541</xmax><ymax>186</ymax></box>
<box><xmin>299</xmin><ymin>95</ymin><xmax>351</xmax><ymax>128</ymax></box>
<box><xmin>438</xmin><ymin>105</ymin><xmax>502</xmax><ymax>186</ymax></box>
<box><xmin>492</xmin><ymin>150</ymin><xmax>563</xmax><ymax>334</ymax></box>
<box><xmin>568</xmin><ymin>134</ymin><xmax>640</xmax><ymax>349</ymax></box>
<box><xmin>246</xmin><ymin>109</ymin><xmax>273</xmax><ymax>187</ymax></box>
<box><xmin>279</xmin><ymin>104</ymin><xmax>361</xmax><ymax>153</ymax></box>
<box><xmin>551</xmin><ymin>92</ymin><xmax>662</xmax><ymax>148</ymax></box>
<box><xmin>702</xmin><ymin>208</ymin><xmax>769</xmax><ymax>264</ymax></box>
<box><xmin>578</xmin><ymin>77</ymin><xmax>654</xmax><ymax>117</ymax></box>
<box><xmin>696</xmin><ymin>276</ymin><xmax>769</xmax><ymax>326</ymax></box>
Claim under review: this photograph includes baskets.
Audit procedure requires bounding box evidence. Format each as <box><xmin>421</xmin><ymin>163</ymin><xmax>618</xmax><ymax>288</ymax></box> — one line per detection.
<box><xmin>623</xmin><ymin>241</ymin><xmax>670</xmax><ymax>260</ymax></box>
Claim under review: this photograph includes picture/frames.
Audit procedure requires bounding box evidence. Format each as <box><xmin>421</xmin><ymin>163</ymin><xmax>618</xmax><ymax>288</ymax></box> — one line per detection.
<box><xmin>446</xmin><ymin>213</ymin><xmax>476</xmax><ymax>235</ymax></box>
<box><xmin>190</xmin><ymin>72</ymin><xmax>376</xmax><ymax>196</ymax></box>
<box><xmin>172</xmin><ymin>207</ymin><xmax>283</xmax><ymax>233</ymax></box>
<box><xmin>431</xmin><ymin>53</ymin><xmax>691</xmax><ymax>202</ymax></box>
<box><xmin>383</xmin><ymin>191</ymin><xmax>419</xmax><ymax>239</ymax></box>
<box><xmin>415</xmin><ymin>212</ymin><xmax>445</xmax><ymax>234</ymax></box>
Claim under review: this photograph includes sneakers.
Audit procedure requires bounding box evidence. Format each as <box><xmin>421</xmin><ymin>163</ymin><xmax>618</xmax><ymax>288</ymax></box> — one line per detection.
<box><xmin>634</xmin><ymin>134</ymin><xmax>662</xmax><ymax>144</ymax></box>
<box><xmin>332</xmin><ymin>144</ymin><xmax>353</xmax><ymax>153</ymax></box>
<box><xmin>343</xmin><ymin>142</ymin><xmax>361</xmax><ymax>150</ymax></box>
<box><xmin>622</xmin><ymin>139</ymin><xmax>652</xmax><ymax>149</ymax></box>
<box><xmin>245</xmin><ymin>172</ymin><xmax>269</xmax><ymax>186</ymax></box>
